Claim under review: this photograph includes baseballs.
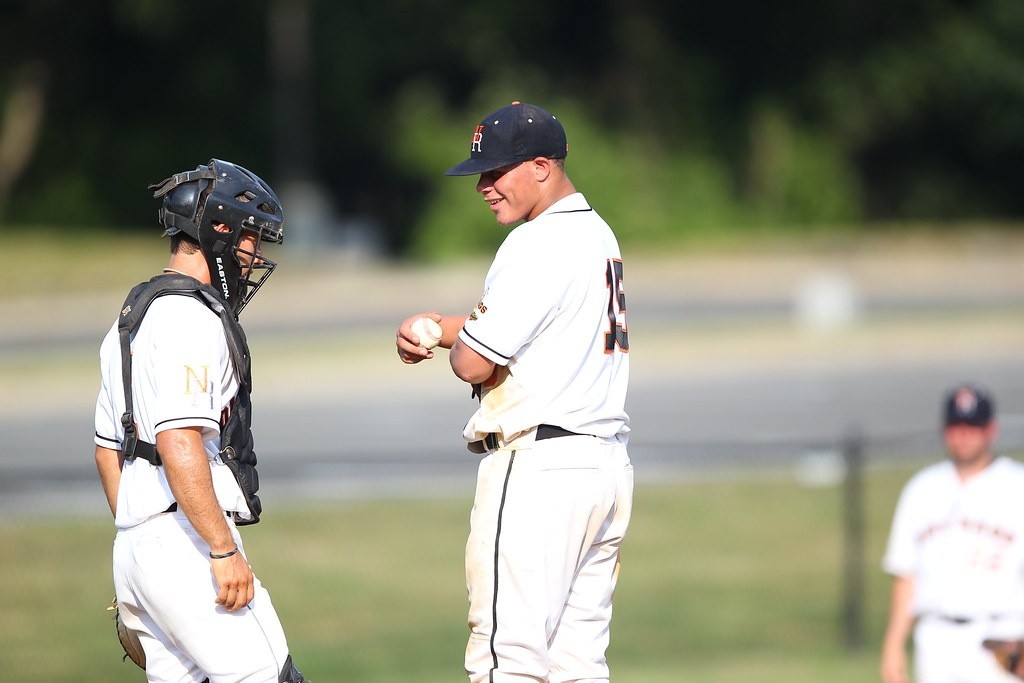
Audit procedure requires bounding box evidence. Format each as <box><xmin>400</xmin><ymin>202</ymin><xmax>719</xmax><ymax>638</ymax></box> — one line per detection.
<box><xmin>411</xmin><ymin>318</ymin><xmax>442</xmax><ymax>350</ymax></box>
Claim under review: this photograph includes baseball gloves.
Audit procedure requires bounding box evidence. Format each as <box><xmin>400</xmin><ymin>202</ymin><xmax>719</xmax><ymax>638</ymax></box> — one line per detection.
<box><xmin>107</xmin><ymin>596</ymin><xmax>147</xmax><ymax>671</ymax></box>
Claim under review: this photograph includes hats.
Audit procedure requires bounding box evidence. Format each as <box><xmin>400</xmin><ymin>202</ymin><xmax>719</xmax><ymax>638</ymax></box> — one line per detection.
<box><xmin>444</xmin><ymin>101</ymin><xmax>569</xmax><ymax>177</ymax></box>
<box><xmin>944</xmin><ymin>385</ymin><xmax>992</xmax><ymax>426</ymax></box>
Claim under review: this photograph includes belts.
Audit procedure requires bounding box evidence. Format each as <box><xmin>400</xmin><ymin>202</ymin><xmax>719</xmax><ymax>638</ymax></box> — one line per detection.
<box><xmin>161</xmin><ymin>502</ymin><xmax>235</xmax><ymax>517</ymax></box>
<box><xmin>944</xmin><ymin>617</ymin><xmax>970</xmax><ymax>624</ymax></box>
<box><xmin>467</xmin><ymin>424</ymin><xmax>580</xmax><ymax>454</ymax></box>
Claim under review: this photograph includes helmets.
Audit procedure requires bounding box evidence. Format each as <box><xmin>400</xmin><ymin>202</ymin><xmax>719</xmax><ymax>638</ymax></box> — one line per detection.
<box><xmin>146</xmin><ymin>158</ymin><xmax>284</xmax><ymax>323</ymax></box>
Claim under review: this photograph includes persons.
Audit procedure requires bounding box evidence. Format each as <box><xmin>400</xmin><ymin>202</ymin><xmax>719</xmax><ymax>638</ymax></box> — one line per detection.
<box><xmin>395</xmin><ymin>101</ymin><xmax>634</xmax><ymax>683</ymax></box>
<box><xmin>92</xmin><ymin>158</ymin><xmax>303</xmax><ymax>683</ymax></box>
<box><xmin>879</xmin><ymin>387</ymin><xmax>1024</xmax><ymax>683</ymax></box>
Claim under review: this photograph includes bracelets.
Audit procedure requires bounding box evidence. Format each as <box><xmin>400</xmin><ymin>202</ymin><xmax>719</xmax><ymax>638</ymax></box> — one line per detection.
<box><xmin>209</xmin><ymin>543</ymin><xmax>238</xmax><ymax>559</ymax></box>
